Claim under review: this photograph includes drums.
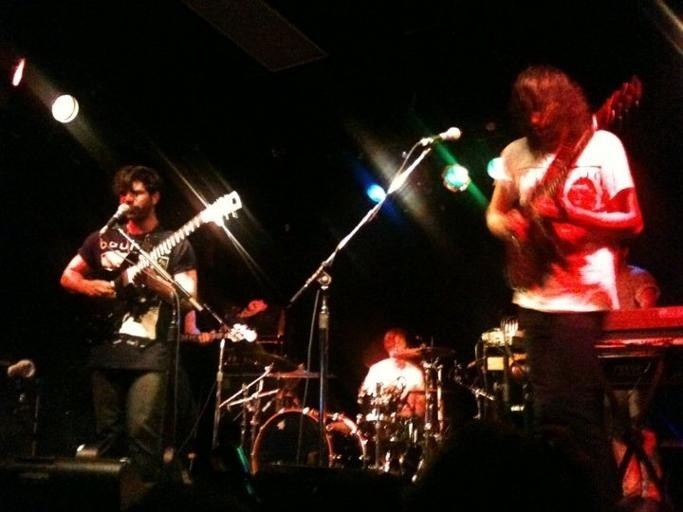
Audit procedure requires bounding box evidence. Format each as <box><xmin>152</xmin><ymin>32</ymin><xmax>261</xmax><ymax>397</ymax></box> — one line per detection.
<box><xmin>361</xmin><ymin>394</ymin><xmax>406</xmax><ymax>443</ymax></box>
<box><xmin>251</xmin><ymin>408</ymin><xmax>369</xmax><ymax>474</ymax></box>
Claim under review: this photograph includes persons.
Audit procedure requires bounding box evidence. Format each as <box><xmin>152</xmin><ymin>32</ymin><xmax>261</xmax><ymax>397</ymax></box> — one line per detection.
<box><xmin>482</xmin><ymin>70</ymin><xmax>647</xmax><ymax>509</ymax></box>
<box><xmin>359</xmin><ymin>331</ymin><xmax>424</xmax><ymax>419</ymax></box>
<box><xmin>607</xmin><ymin>237</ymin><xmax>664</xmax><ymax>502</ymax></box>
<box><xmin>60</xmin><ymin>166</ymin><xmax>211</xmax><ymax>469</ymax></box>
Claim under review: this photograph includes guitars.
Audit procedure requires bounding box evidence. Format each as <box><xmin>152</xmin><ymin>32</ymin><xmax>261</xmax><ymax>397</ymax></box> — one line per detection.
<box><xmin>75</xmin><ymin>191</ymin><xmax>243</xmax><ymax>342</ymax></box>
<box><xmin>174</xmin><ymin>324</ymin><xmax>257</xmax><ymax>344</ymax></box>
<box><xmin>503</xmin><ymin>74</ymin><xmax>642</xmax><ymax>291</ymax></box>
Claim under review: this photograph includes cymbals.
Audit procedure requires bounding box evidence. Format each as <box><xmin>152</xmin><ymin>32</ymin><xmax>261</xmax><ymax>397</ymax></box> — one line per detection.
<box><xmin>272</xmin><ymin>369</ymin><xmax>337</xmax><ymax>379</ymax></box>
<box><xmin>419</xmin><ymin>348</ymin><xmax>456</xmax><ymax>356</ymax></box>
<box><xmin>243</xmin><ymin>351</ymin><xmax>299</xmax><ymax>373</ymax></box>
<box><xmin>407</xmin><ymin>389</ymin><xmax>449</xmax><ymax>395</ymax></box>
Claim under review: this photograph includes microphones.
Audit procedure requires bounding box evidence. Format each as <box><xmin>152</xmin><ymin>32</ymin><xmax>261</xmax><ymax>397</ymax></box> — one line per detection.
<box><xmin>97</xmin><ymin>203</ymin><xmax>131</xmax><ymax>236</ymax></box>
<box><xmin>419</xmin><ymin>126</ymin><xmax>462</xmax><ymax>147</ymax></box>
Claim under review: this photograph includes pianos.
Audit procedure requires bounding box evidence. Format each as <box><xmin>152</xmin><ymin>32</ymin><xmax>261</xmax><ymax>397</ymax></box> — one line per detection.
<box><xmin>597</xmin><ymin>307</ymin><xmax>680</xmax><ymax>351</ymax></box>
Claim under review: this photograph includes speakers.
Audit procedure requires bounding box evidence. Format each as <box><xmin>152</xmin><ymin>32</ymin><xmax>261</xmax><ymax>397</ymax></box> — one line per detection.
<box><xmin>252</xmin><ymin>465</ymin><xmax>401</xmax><ymax>511</ymax></box>
<box><xmin>0</xmin><ymin>456</ymin><xmax>145</xmax><ymax>512</ymax></box>
<box><xmin>421</xmin><ymin>416</ymin><xmax>624</xmax><ymax>511</ymax></box>
